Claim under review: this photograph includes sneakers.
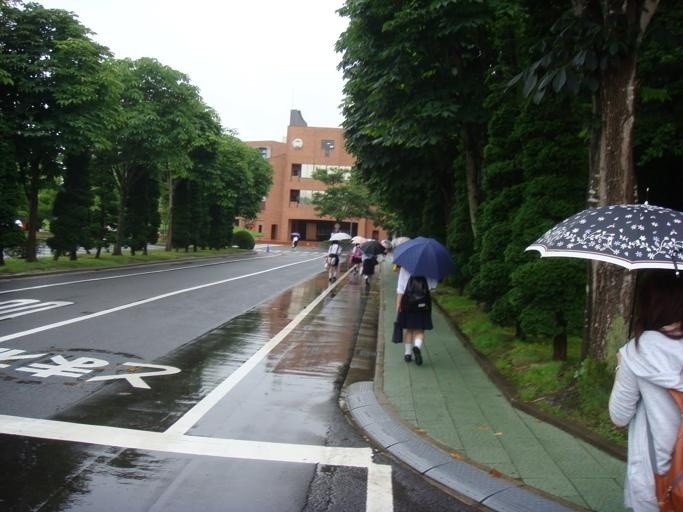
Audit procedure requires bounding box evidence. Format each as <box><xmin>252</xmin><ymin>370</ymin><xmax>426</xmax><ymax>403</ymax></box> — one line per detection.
<box><xmin>328</xmin><ymin>270</ymin><xmax>370</xmax><ymax>286</ymax></box>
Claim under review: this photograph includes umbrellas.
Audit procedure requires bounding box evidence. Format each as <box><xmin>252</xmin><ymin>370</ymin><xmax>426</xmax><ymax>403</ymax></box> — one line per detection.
<box><xmin>327</xmin><ymin>231</ymin><xmax>457</xmax><ymax>283</ymax></box>
<box><xmin>291</xmin><ymin>233</ymin><xmax>300</xmax><ymax>235</ymax></box>
<box><xmin>524</xmin><ymin>186</ymin><xmax>683</xmax><ymax>341</ymax></box>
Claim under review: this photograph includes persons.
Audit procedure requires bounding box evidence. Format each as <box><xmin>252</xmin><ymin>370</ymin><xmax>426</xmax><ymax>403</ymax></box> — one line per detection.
<box><xmin>396</xmin><ymin>267</ymin><xmax>438</xmax><ymax>366</ymax></box>
<box><xmin>607</xmin><ymin>268</ymin><xmax>682</xmax><ymax>511</ymax></box>
<box><xmin>291</xmin><ymin>236</ymin><xmax>299</xmax><ymax>249</ymax></box>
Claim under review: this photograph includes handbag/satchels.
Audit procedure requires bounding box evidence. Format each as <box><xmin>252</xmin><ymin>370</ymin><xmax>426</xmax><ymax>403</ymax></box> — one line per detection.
<box><xmin>324</xmin><ymin>256</ymin><xmax>336</xmax><ymax>266</ymax></box>
<box><xmin>392</xmin><ymin>311</ymin><xmax>404</xmax><ymax>343</ymax></box>
<box><xmin>324</xmin><ymin>263</ymin><xmax>329</xmax><ymax>269</ymax></box>
<box><xmin>391</xmin><ymin>264</ymin><xmax>400</xmax><ymax>272</ymax></box>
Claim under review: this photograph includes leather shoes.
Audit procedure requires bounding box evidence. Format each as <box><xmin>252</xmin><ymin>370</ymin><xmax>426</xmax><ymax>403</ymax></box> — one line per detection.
<box><xmin>413</xmin><ymin>346</ymin><xmax>423</xmax><ymax>366</ymax></box>
<box><xmin>404</xmin><ymin>354</ymin><xmax>412</xmax><ymax>362</ymax></box>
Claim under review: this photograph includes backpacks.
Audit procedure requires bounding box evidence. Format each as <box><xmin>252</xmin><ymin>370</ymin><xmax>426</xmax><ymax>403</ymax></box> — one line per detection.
<box><xmin>653</xmin><ymin>388</ymin><xmax>683</xmax><ymax>512</ymax></box>
<box><xmin>401</xmin><ymin>275</ymin><xmax>432</xmax><ymax>314</ymax></box>
